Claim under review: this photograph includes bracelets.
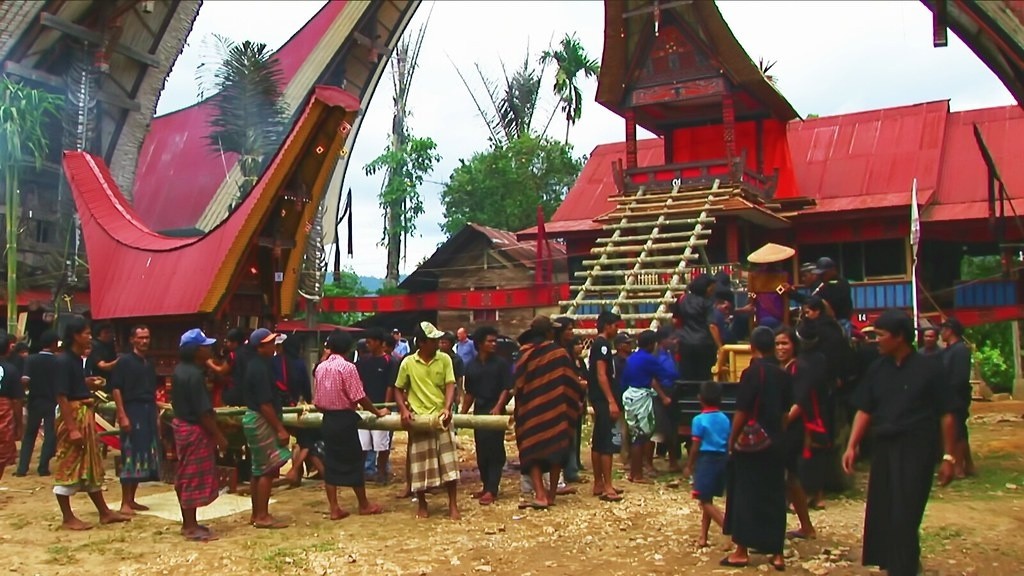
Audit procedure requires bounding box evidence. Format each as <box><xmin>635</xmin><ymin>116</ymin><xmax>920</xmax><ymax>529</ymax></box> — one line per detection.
<box><xmin>942</xmin><ymin>453</ymin><xmax>956</xmax><ymax>464</ymax></box>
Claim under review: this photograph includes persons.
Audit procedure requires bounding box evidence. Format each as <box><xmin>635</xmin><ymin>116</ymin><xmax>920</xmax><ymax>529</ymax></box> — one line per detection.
<box><xmin>0</xmin><ymin>243</ymin><xmax>973</xmax><ymax>576</ymax></box>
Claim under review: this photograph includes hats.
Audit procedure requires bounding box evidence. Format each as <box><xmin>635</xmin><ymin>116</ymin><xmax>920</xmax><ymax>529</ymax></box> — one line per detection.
<box><xmin>940</xmin><ymin>315</ymin><xmax>965</xmax><ymax>336</ymax></box>
<box><xmin>415</xmin><ymin>321</ymin><xmax>446</xmax><ymax>340</ymax></box>
<box><xmin>179</xmin><ymin>328</ymin><xmax>217</xmax><ymax>347</ymax></box>
<box><xmin>747</xmin><ymin>242</ymin><xmax>796</xmax><ymax>263</ymax></box>
<box><xmin>250</xmin><ymin>328</ymin><xmax>281</xmax><ymax>345</ymax></box>
<box><xmin>531</xmin><ymin>314</ymin><xmax>562</xmax><ymax>328</ymax></box>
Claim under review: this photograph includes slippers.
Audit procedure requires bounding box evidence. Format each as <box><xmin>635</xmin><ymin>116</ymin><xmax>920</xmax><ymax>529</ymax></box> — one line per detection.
<box><xmin>769</xmin><ymin>556</ymin><xmax>785</xmax><ymax>571</ymax></box>
<box><xmin>721</xmin><ymin>556</ymin><xmax>748</xmax><ymax>567</ymax></box>
<box><xmin>186</xmin><ymin>525</ymin><xmax>220</xmax><ymax>541</ymax></box>
<box><xmin>253</xmin><ymin>517</ymin><xmax>289</xmax><ymax>528</ymax></box>
